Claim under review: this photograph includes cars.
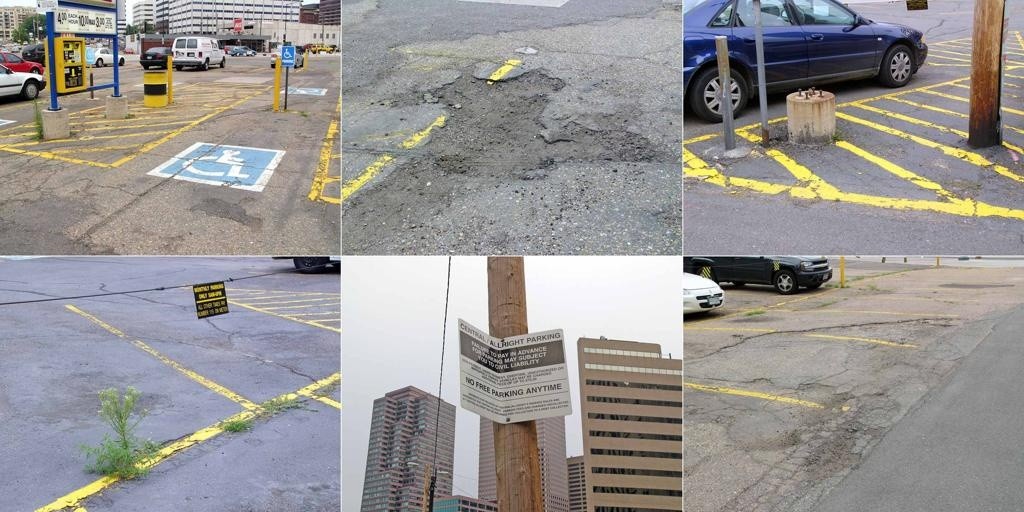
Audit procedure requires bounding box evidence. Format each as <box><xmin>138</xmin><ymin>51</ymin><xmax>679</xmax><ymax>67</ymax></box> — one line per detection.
<box><xmin>0</xmin><ymin>41</ymin><xmax>28</xmax><ymax>53</ymax></box>
<box><xmin>85</xmin><ymin>47</ymin><xmax>126</xmax><ymax>69</ymax></box>
<box><xmin>138</xmin><ymin>47</ymin><xmax>172</xmax><ymax>71</ymax></box>
<box><xmin>271</xmin><ymin>256</ymin><xmax>340</xmax><ymax>274</ymax></box>
<box><xmin>682</xmin><ymin>272</ymin><xmax>727</xmax><ymax>320</ymax></box>
<box><xmin>683</xmin><ymin>0</ymin><xmax>929</xmax><ymax>126</ymax></box>
<box><xmin>0</xmin><ymin>64</ymin><xmax>47</xmax><ymax>103</ymax></box>
<box><xmin>270</xmin><ymin>46</ymin><xmax>303</xmax><ymax>70</ymax></box>
<box><xmin>223</xmin><ymin>44</ymin><xmax>257</xmax><ymax>56</ymax></box>
<box><xmin>85</xmin><ymin>40</ymin><xmax>134</xmax><ymax>55</ymax></box>
<box><xmin>0</xmin><ymin>49</ymin><xmax>44</xmax><ymax>75</ymax></box>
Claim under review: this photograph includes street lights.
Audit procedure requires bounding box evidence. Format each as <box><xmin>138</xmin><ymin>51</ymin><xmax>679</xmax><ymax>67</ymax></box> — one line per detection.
<box><xmin>284</xmin><ymin>2</ymin><xmax>292</xmax><ymax>43</ymax></box>
<box><xmin>313</xmin><ymin>12</ymin><xmax>324</xmax><ymax>42</ymax></box>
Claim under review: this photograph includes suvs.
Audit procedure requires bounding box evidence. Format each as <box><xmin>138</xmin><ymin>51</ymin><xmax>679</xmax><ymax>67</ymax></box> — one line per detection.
<box><xmin>19</xmin><ymin>44</ymin><xmax>44</xmax><ymax>67</ymax></box>
<box><xmin>682</xmin><ymin>256</ymin><xmax>834</xmax><ymax>296</ymax></box>
<box><xmin>310</xmin><ymin>45</ymin><xmax>333</xmax><ymax>55</ymax></box>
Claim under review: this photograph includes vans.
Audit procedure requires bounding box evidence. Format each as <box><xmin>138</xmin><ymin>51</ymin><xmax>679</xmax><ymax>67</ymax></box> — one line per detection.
<box><xmin>169</xmin><ymin>37</ymin><xmax>228</xmax><ymax>71</ymax></box>
